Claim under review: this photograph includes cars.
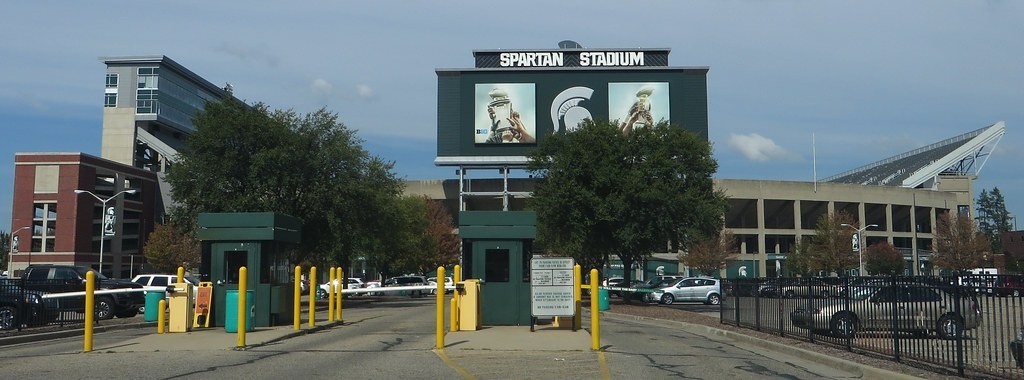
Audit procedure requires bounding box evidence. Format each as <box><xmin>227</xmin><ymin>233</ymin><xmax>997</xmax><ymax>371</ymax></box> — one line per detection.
<box><xmin>0</xmin><ymin>276</ymin><xmax>59</xmax><ymax>329</ymax></box>
<box><xmin>789</xmin><ymin>281</ymin><xmax>981</xmax><ymax>339</ymax></box>
<box><xmin>749</xmin><ymin>275</ymin><xmax>877</xmax><ymax>298</ymax></box>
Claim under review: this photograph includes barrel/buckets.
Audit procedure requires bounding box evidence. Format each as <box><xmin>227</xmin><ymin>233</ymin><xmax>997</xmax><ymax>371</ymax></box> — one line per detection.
<box><xmin>225</xmin><ymin>290</ymin><xmax>256</xmax><ymax>331</ymax></box>
<box><xmin>145</xmin><ymin>290</ymin><xmax>166</xmax><ymax>321</ymax></box>
<box><xmin>598</xmin><ymin>289</ymin><xmax>609</xmax><ymax>310</ymax></box>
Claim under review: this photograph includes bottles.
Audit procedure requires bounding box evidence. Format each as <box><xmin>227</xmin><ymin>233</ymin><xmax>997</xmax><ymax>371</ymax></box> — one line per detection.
<box><xmin>487</xmin><ymin>88</ymin><xmax>513</xmax><ymax>131</ymax></box>
<box><xmin>634</xmin><ymin>87</ymin><xmax>653</xmax><ymax>124</ymax></box>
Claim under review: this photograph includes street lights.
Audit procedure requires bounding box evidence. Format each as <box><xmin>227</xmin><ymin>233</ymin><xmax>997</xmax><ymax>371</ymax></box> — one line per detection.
<box><xmin>8</xmin><ymin>226</ymin><xmax>32</xmax><ymax>278</ymax></box>
<box><xmin>74</xmin><ymin>188</ymin><xmax>138</xmax><ymax>274</ymax></box>
<box><xmin>841</xmin><ymin>223</ymin><xmax>878</xmax><ymax>275</ymax></box>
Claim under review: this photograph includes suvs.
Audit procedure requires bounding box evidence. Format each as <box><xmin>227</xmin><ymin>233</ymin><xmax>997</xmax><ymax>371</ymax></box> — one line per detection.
<box><xmin>18</xmin><ymin>261</ymin><xmax>147</xmax><ymax>319</ymax></box>
<box><xmin>129</xmin><ymin>272</ymin><xmax>200</xmax><ymax>315</ymax></box>
<box><xmin>980</xmin><ymin>279</ymin><xmax>997</xmax><ymax>295</ymax></box>
<box><xmin>995</xmin><ymin>273</ymin><xmax>1023</xmax><ymax>296</ymax></box>
<box><xmin>602</xmin><ymin>274</ymin><xmax>734</xmax><ymax>305</ymax></box>
<box><xmin>319</xmin><ymin>274</ymin><xmax>453</xmax><ymax>298</ymax></box>
<box><xmin>299</xmin><ymin>280</ymin><xmax>310</xmax><ymax>293</ymax></box>
